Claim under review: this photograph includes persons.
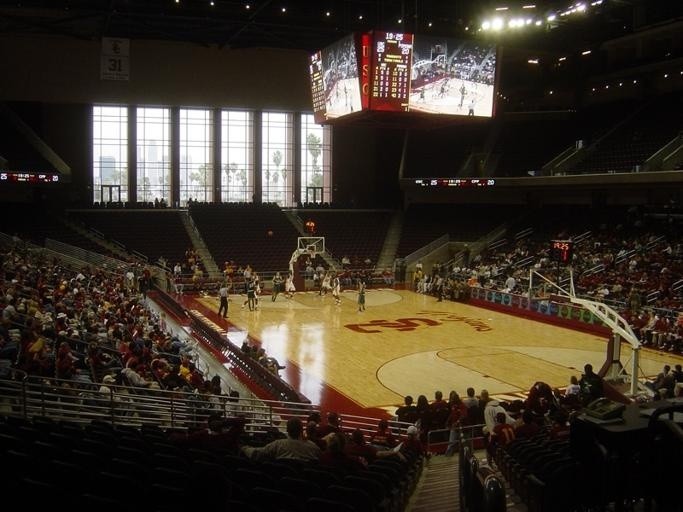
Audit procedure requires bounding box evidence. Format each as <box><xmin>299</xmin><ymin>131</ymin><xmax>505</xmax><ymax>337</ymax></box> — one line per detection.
<box><xmin>413</xmin><ymin>45</ymin><xmax>496</xmax><ymax>116</ymax></box>
<box><xmin>322</xmin><ymin>38</ymin><xmax>360</xmax><ymax>113</ymax></box>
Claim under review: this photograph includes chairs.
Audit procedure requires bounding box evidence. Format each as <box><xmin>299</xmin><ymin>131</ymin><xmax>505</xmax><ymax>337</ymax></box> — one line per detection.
<box><xmin>0</xmin><ymin>412</ymin><xmax>420</xmax><ymax>512</ymax></box>
<box><xmin>494</xmin><ymin>422</ymin><xmax>625</xmax><ymax>512</ymax></box>
<box><xmin>1</xmin><ymin>202</ymin><xmax>602</xmax><ymax>275</ymax></box>
<box><xmin>419</xmin><ymin>91</ymin><xmax>683</xmax><ymax>174</ymax></box>
